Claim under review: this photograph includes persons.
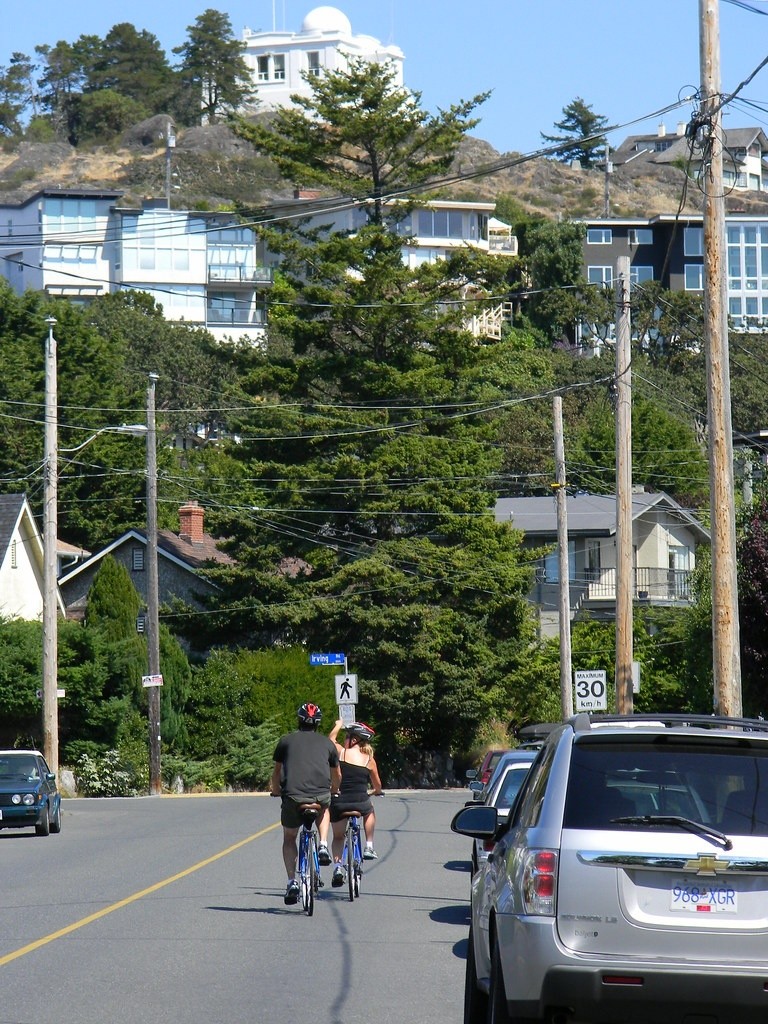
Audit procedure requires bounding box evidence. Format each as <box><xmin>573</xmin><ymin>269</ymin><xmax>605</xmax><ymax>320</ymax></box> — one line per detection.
<box><xmin>326</xmin><ymin>715</ymin><xmax>381</xmax><ymax>887</ymax></box>
<box><xmin>270</xmin><ymin>702</ymin><xmax>343</xmax><ymax>905</ymax></box>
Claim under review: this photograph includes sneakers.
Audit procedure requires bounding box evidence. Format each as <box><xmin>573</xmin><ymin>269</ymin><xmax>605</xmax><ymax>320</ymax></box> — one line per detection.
<box><xmin>331</xmin><ymin>866</ymin><xmax>345</xmax><ymax>888</ymax></box>
<box><xmin>284</xmin><ymin>879</ymin><xmax>300</xmax><ymax>905</ymax></box>
<box><xmin>318</xmin><ymin>845</ymin><xmax>332</xmax><ymax>866</ymax></box>
<box><xmin>363</xmin><ymin>847</ymin><xmax>378</xmax><ymax>859</ymax></box>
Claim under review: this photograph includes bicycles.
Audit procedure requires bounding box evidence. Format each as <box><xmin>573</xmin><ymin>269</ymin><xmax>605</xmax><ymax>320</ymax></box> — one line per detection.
<box><xmin>270</xmin><ymin>789</ymin><xmax>338</xmax><ymax>915</ymax></box>
<box><xmin>326</xmin><ymin>791</ymin><xmax>386</xmax><ymax>901</ymax></box>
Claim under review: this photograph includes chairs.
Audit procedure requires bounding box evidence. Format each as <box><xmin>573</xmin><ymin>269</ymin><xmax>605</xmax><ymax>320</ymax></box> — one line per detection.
<box><xmin>579</xmin><ymin>786</ymin><xmax>767</xmax><ymax>831</ymax></box>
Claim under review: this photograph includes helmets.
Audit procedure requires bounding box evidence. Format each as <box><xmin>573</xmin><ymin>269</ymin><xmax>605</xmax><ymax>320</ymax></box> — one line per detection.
<box><xmin>297</xmin><ymin>703</ymin><xmax>322</xmax><ymax>728</ymax></box>
<box><xmin>344</xmin><ymin>722</ymin><xmax>375</xmax><ymax>743</ymax></box>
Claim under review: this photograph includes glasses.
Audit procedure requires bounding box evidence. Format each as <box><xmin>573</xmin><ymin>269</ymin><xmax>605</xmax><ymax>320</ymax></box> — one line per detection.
<box><xmin>346</xmin><ymin>734</ymin><xmax>350</xmax><ymax>739</ymax></box>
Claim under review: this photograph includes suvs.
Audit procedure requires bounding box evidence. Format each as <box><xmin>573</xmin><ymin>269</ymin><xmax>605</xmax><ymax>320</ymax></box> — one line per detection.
<box><xmin>447</xmin><ymin>717</ymin><xmax>768</xmax><ymax>1022</ymax></box>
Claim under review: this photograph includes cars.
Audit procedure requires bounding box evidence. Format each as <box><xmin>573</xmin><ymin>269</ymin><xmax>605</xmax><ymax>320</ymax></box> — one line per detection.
<box><xmin>593</xmin><ymin>765</ymin><xmax>716</xmax><ymax>829</ymax></box>
<box><xmin>0</xmin><ymin>748</ymin><xmax>62</xmax><ymax>836</ymax></box>
<box><xmin>461</xmin><ymin>747</ymin><xmax>542</xmax><ymax>881</ymax></box>
